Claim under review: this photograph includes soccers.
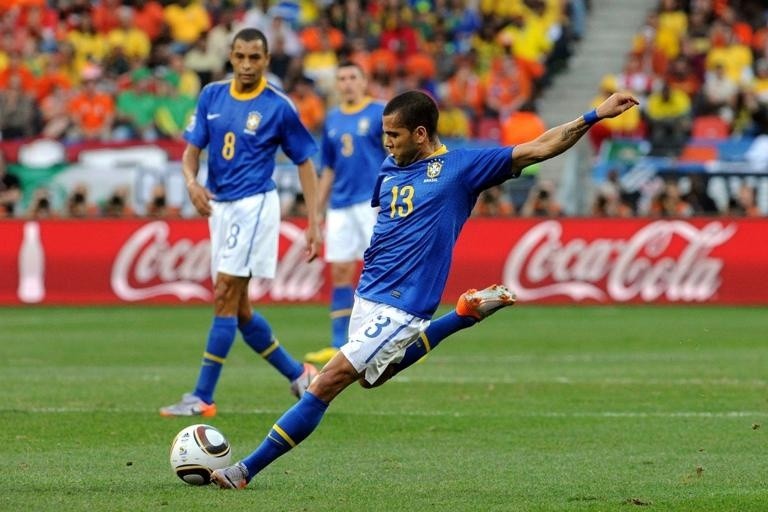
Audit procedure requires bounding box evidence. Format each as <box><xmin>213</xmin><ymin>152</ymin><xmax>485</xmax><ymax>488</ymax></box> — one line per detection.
<box><xmin>170</xmin><ymin>424</ymin><xmax>231</xmax><ymax>485</ymax></box>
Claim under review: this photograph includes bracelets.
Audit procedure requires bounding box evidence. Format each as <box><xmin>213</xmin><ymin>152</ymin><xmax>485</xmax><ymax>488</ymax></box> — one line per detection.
<box><xmin>583</xmin><ymin>109</ymin><xmax>600</xmax><ymax>126</ymax></box>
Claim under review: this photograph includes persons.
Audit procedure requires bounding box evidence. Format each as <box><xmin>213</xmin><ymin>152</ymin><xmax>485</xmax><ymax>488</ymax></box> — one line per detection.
<box><xmin>159</xmin><ymin>27</ymin><xmax>319</xmax><ymax>416</ymax></box>
<box><xmin>212</xmin><ymin>90</ymin><xmax>641</xmax><ymax>489</ymax></box>
<box><xmin>587</xmin><ymin>0</ymin><xmax>767</xmax><ymax>221</ymax></box>
<box><xmin>304</xmin><ymin>62</ymin><xmax>391</xmax><ymax>365</ymax></box>
<box><xmin>1</xmin><ymin>0</ymin><xmax>586</xmax><ymax>220</ymax></box>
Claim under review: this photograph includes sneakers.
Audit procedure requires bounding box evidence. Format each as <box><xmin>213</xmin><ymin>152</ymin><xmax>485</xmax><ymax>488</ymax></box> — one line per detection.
<box><xmin>210</xmin><ymin>463</ymin><xmax>249</xmax><ymax>489</ymax></box>
<box><xmin>290</xmin><ymin>363</ymin><xmax>317</xmax><ymax>399</ymax></box>
<box><xmin>456</xmin><ymin>284</ymin><xmax>518</xmax><ymax>321</ymax></box>
<box><xmin>159</xmin><ymin>393</ymin><xmax>217</xmax><ymax>417</ymax></box>
